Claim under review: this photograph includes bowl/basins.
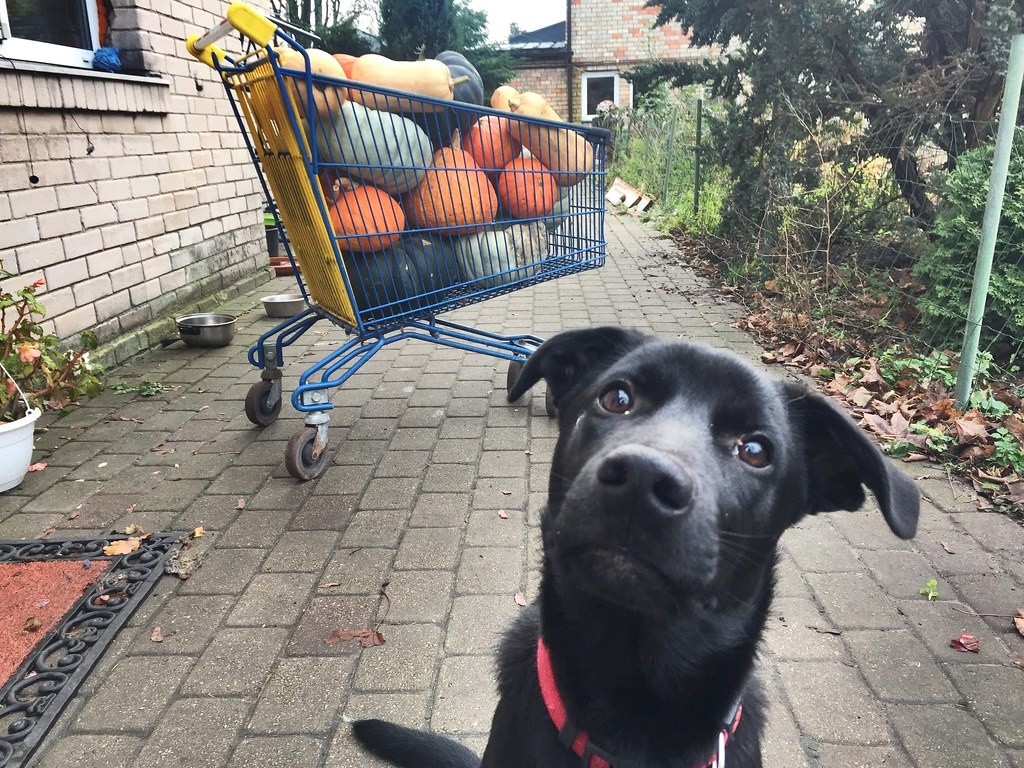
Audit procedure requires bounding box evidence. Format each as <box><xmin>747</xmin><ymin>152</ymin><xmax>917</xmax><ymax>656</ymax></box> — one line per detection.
<box><xmin>260</xmin><ymin>295</ymin><xmax>304</xmax><ymax>318</ymax></box>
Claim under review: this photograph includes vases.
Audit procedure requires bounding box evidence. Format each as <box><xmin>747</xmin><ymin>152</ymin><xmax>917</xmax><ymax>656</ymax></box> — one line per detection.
<box><xmin>0</xmin><ymin>364</ymin><xmax>42</xmax><ymax>493</ymax></box>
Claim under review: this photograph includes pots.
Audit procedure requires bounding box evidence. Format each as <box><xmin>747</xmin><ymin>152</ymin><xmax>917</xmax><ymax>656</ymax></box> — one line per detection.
<box><xmin>173</xmin><ymin>311</ymin><xmax>250</xmax><ymax>347</ymax></box>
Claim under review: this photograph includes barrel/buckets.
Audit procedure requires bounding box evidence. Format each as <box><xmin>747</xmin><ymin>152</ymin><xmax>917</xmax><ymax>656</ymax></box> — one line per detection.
<box><xmin>265</xmin><ymin>225</ymin><xmax>281</xmax><ymax>266</ymax></box>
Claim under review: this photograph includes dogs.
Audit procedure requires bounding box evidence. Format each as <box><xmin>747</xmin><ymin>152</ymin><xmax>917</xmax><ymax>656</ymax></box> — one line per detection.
<box><xmin>350</xmin><ymin>326</ymin><xmax>921</xmax><ymax>768</ymax></box>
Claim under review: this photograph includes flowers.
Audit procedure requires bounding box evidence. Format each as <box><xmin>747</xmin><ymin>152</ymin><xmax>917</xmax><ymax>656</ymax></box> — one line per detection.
<box><xmin>0</xmin><ymin>259</ymin><xmax>106</xmax><ymax>424</ymax></box>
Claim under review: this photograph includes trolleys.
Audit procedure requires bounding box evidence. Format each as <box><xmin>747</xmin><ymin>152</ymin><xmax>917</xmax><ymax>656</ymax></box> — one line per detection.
<box><xmin>186</xmin><ymin>2</ymin><xmax>612</xmax><ymax>481</ymax></box>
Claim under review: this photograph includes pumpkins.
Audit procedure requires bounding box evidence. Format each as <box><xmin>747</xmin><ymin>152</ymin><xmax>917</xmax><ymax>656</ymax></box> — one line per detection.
<box><xmin>248</xmin><ymin>47</ymin><xmax>594</xmax><ymax>317</ymax></box>
